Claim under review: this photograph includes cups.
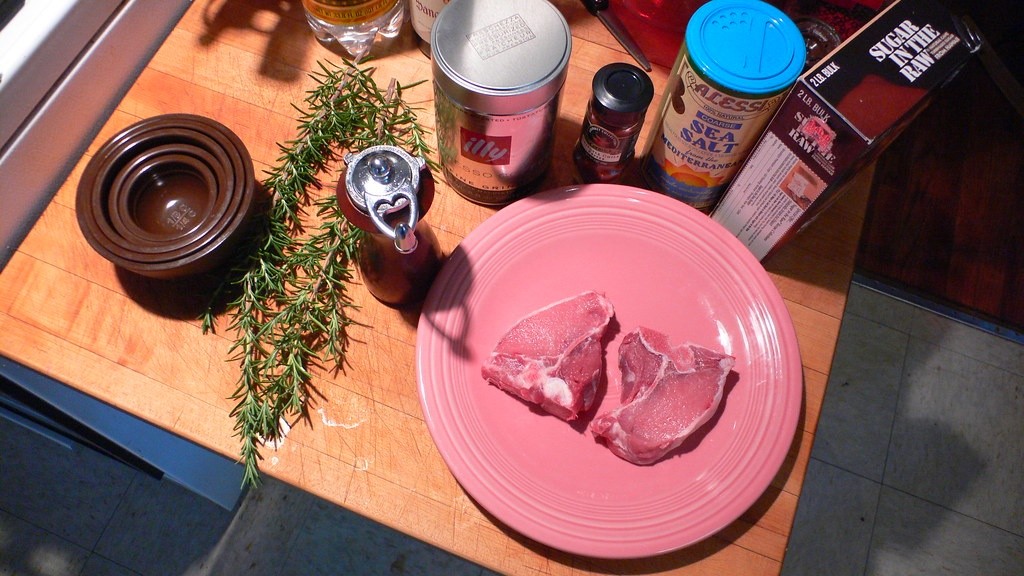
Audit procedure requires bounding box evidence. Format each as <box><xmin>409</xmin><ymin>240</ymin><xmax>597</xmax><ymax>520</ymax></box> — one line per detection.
<box><xmin>793</xmin><ymin>16</ymin><xmax>843</xmax><ymax>73</ymax></box>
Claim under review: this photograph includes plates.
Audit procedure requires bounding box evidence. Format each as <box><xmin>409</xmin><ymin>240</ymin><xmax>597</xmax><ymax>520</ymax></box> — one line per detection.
<box><xmin>414</xmin><ymin>183</ymin><xmax>804</xmax><ymax>560</ymax></box>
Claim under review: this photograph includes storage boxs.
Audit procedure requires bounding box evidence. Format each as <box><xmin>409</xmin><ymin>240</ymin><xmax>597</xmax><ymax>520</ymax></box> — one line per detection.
<box><xmin>710</xmin><ymin>0</ymin><xmax>993</xmax><ymax>265</ymax></box>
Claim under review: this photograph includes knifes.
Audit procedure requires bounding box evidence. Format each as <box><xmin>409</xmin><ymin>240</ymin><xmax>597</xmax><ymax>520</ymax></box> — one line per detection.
<box><xmin>580</xmin><ymin>0</ymin><xmax>651</xmax><ymax>71</ymax></box>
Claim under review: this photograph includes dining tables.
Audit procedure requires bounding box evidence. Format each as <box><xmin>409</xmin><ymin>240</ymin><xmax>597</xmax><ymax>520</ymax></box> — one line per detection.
<box><xmin>0</xmin><ymin>0</ymin><xmax>878</xmax><ymax>575</ymax></box>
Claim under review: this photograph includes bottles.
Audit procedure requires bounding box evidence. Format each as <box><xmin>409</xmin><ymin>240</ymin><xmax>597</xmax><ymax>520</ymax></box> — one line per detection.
<box><xmin>408</xmin><ymin>0</ymin><xmax>451</xmax><ymax>60</ymax></box>
<box><xmin>573</xmin><ymin>62</ymin><xmax>654</xmax><ymax>188</ymax></box>
<box><xmin>301</xmin><ymin>0</ymin><xmax>405</xmax><ymax>58</ymax></box>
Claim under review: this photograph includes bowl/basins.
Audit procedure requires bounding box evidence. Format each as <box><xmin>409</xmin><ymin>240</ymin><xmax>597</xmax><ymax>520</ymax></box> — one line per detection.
<box><xmin>73</xmin><ymin>113</ymin><xmax>257</xmax><ymax>284</ymax></box>
